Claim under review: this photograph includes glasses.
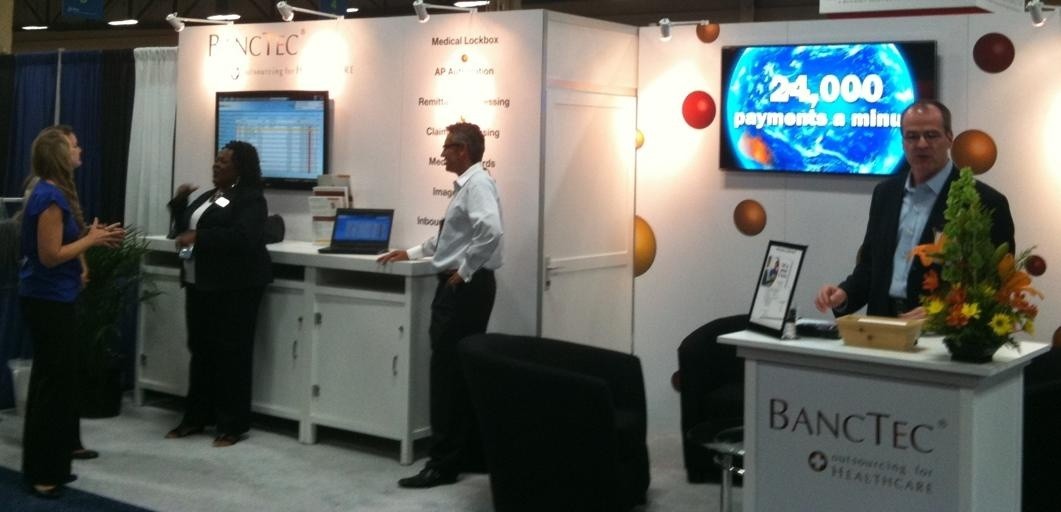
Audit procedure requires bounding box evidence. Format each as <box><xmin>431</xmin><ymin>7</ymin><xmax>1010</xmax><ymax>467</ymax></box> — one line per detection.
<box><xmin>442</xmin><ymin>143</ymin><xmax>458</xmax><ymax>148</ymax></box>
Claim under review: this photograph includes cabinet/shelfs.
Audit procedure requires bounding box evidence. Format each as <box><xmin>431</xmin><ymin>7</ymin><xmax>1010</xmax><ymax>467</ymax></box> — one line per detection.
<box><xmin>304</xmin><ymin>257</ymin><xmax>437</xmax><ymax>464</ymax></box>
<box><xmin>136</xmin><ymin>239</ymin><xmax>191</xmax><ymax>410</ymax></box>
<box><xmin>251</xmin><ymin>252</ymin><xmax>303</xmax><ymax>446</ymax></box>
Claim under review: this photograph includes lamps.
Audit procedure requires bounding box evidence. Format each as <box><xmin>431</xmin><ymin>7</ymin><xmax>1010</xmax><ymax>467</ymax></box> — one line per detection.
<box><xmin>1023</xmin><ymin>1</ymin><xmax>1046</xmax><ymax>26</ymax></box>
<box><xmin>414</xmin><ymin>0</ymin><xmax>477</xmax><ymax>22</ymax></box>
<box><xmin>277</xmin><ymin>1</ymin><xmax>344</xmax><ymax>22</ymax></box>
<box><xmin>166</xmin><ymin>15</ymin><xmax>234</xmax><ymax>31</ymax></box>
<box><xmin>660</xmin><ymin>18</ymin><xmax>709</xmax><ymax>41</ymax></box>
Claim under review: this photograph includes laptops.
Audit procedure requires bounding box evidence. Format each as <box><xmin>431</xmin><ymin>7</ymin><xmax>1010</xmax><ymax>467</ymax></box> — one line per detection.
<box><xmin>317</xmin><ymin>208</ymin><xmax>394</xmax><ymax>253</ymax></box>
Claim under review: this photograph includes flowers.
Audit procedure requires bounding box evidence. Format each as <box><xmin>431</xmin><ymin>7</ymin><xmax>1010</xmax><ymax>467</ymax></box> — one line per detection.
<box><xmin>906</xmin><ymin>167</ymin><xmax>1045</xmax><ymax>354</ymax></box>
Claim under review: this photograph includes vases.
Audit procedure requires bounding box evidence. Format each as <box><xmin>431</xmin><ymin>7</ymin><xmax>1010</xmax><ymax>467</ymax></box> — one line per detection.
<box><xmin>944</xmin><ymin>331</ymin><xmax>999</xmax><ymax>362</ymax></box>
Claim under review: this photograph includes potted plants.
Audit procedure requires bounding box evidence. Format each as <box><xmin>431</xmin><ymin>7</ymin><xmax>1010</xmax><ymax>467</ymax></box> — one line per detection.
<box><xmin>73</xmin><ymin>224</ymin><xmax>164</xmax><ymax>417</ymax></box>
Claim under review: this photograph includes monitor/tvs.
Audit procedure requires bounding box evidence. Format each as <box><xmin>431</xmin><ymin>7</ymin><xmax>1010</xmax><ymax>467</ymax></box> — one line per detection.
<box><xmin>719</xmin><ymin>40</ymin><xmax>939</xmax><ymax>179</ymax></box>
<box><xmin>213</xmin><ymin>89</ymin><xmax>331</xmax><ymax>192</ymax></box>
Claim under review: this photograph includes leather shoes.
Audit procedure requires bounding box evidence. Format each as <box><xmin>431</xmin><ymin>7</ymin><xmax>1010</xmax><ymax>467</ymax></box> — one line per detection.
<box><xmin>164</xmin><ymin>423</ymin><xmax>198</xmax><ymax>438</ymax></box>
<box><xmin>70</xmin><ymin>447</ymin><xmax>99</xmax><ymax>459</ymax></box>
<box><xmin>398</xmin><ymin>463</ymin><xmax>458</xmax><ymax>488</ymax></box>
<box><xmin>211</xmin><ymin>433</ymin><xmax>238</xmax><ymax>448</ymax></box>
<box><xmin>29</xmin><ymin>481</ymin><xmax>64</xmax><ymax>500</ymax></box>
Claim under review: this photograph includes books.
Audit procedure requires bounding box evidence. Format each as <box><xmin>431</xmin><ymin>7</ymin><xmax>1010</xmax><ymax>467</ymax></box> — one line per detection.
<box><xmin>308</xmin><ymin>174</ymin><xmax>351</xmax><ymax>244</ymax></box>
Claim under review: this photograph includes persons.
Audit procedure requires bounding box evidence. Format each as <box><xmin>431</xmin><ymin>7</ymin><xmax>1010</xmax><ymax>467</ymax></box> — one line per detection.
<box><xmin>164</xmin><ymin>140</ymin><xmax>270</xmax><ymax>447</ymax></box>
<box><xmin>377</xmin><ymin>123</ymin><xmax>504</xmax><ymax>489</ymax></box>
<box><xmin>17</xmin><ymin>125</ymin><xmax>125</xmax><ymax>499</ymax></box>
<box><xmin>814</xmin><ymin>102</ymin><xmax>1016</xmax><ymax>326</ymax></box>
<box><xmin>23</xmin><ymin>126</ymin><xmax>91</xmax><ymax>292</ymax></box>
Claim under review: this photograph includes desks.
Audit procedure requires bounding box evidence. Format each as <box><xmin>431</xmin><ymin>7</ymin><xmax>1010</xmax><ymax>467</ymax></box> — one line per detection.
<box><xmin>673</xmin><ymin>308</ymin><xmax>796</xmax><ymax>511</ymax></box>
<box><xmin>470</xmin><ymin>334</ymin><xmax>649</xmax><ymax>511</ymax></box>
